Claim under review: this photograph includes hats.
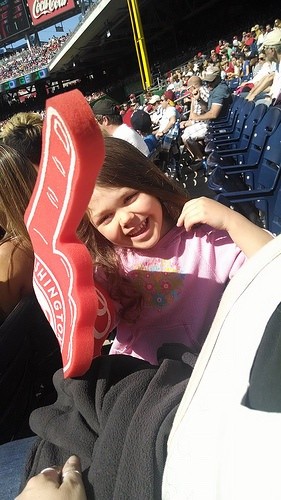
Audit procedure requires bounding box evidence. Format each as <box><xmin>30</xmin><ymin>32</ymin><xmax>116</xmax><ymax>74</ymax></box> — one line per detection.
<box><xmin>263</xmin><ymin>30</ymin><xmax>281</xmax><ymax>44</ymax></box>
<box><xmin>148</xmin><ymin>95</ymin><xmax>160</xmax><ymax>104</ymax></box>
<box><xmin>235</xmin><ymin>53</ymin><xmax>245</xmax><ymax>58</ymax></box>
<box><xmin>92</xmin><ymin>99</ymin><xmax>119</xmax><ymax>115</ymax></box>
<box><xmin>246</xmin><ymin>52</ymin><xmax>258</xmax><ymax>60</ymax></box>
<box><xmin>202</xmin><ymin>66</ymin><xmax>221</xmax><ymax>81</ymax></box>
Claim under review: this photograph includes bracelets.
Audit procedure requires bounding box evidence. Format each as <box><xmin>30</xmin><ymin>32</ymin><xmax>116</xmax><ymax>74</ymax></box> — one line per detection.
<box><xmin>183</xmin><ymin>121</ymin><xmax>187</xmax><ymax>128</ymax></box>
<box><xmin>162</xmin><ymin>131</ymin><xmax>165</xmax><ymax>134</ymax></box>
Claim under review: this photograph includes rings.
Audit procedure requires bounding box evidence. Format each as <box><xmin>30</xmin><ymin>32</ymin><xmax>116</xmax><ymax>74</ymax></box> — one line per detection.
<box><xmin>40</xmin><ymin>467</ymin><xmax>60</xmax><ymax>475</ymax></box>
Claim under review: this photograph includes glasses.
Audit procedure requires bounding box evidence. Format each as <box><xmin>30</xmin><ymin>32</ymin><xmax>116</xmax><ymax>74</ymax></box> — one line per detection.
<box><xmin>258</xmin><ymin>58</ymin><xmax>264</xmax><ymax>60</ymax></box>
<box><xmin>161</xmin><ymin>100</ymin><xmax>165</xmax><ymax>102</ymax></box>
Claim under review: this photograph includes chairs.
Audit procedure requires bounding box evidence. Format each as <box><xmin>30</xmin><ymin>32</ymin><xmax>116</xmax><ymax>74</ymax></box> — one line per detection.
<box><xmin>0</xmin><ymin>65</ymin><xmax>281</xmax><ymax>446</ymax></box>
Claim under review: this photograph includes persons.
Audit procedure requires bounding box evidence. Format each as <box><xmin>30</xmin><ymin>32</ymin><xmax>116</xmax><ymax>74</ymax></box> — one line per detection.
<box><xmin>0</xmin><ymin>77</ymin><xmax>119</xmax><ymax>132</ymax></box>
<box><xmin>14</xmin><ymin>455</ymin><xmax>87</xmax><ymax>500</ymax></box>
<box><xmin>0</xmin><ymin>112</ymin><xmax>45</xmax><ymax>173</ymax></box>
<box><xmin>0</xmin><ymin>33</ymin><xmax>70</xmax><ymax>83</ymax></box>
<box><xmin>91</xmin><ymin>98</ymin><xmax>151</xmax><ymax>162</ymax></box>
<box><xmin>0</xmin><ymin>143</ymin><xmax>38</xmax><ymax>322</ymax></box>
<box><xmin>76</xmin><ymin>136</ymin><xmax>277</xmax><ymax>367</ymax></box>
<box><xmin>115</xmin><ymin>19</ymin><xmax>281</xmax><ymax>169</ymax></box>
